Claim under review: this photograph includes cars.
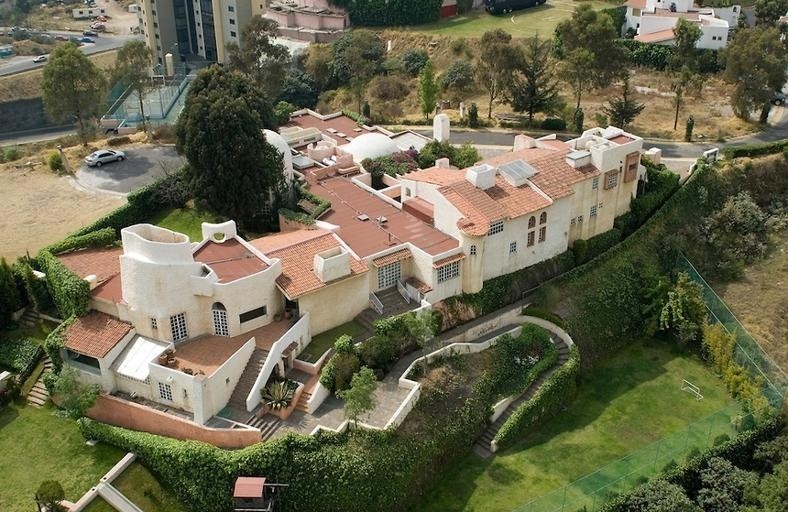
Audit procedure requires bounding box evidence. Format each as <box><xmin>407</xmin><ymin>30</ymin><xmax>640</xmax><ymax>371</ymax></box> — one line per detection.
<box><xmin>85</xmin><ymin>149</ymin><xmax>125</xmax><ymax>167</ymax></box>
<box><xmin>33</xmin><ymin>56</ymin><xmax>46</xmax><ymax>63</ymax></box>
<box><xmin>78</xmin><ymin>29</ymin><xmax>96</xmax><ymax>43</ymax></box>
<box><xmin>41</xmin><ymin>33</ymin><xmax>68</xmax><ymax>41</ymax></box>
